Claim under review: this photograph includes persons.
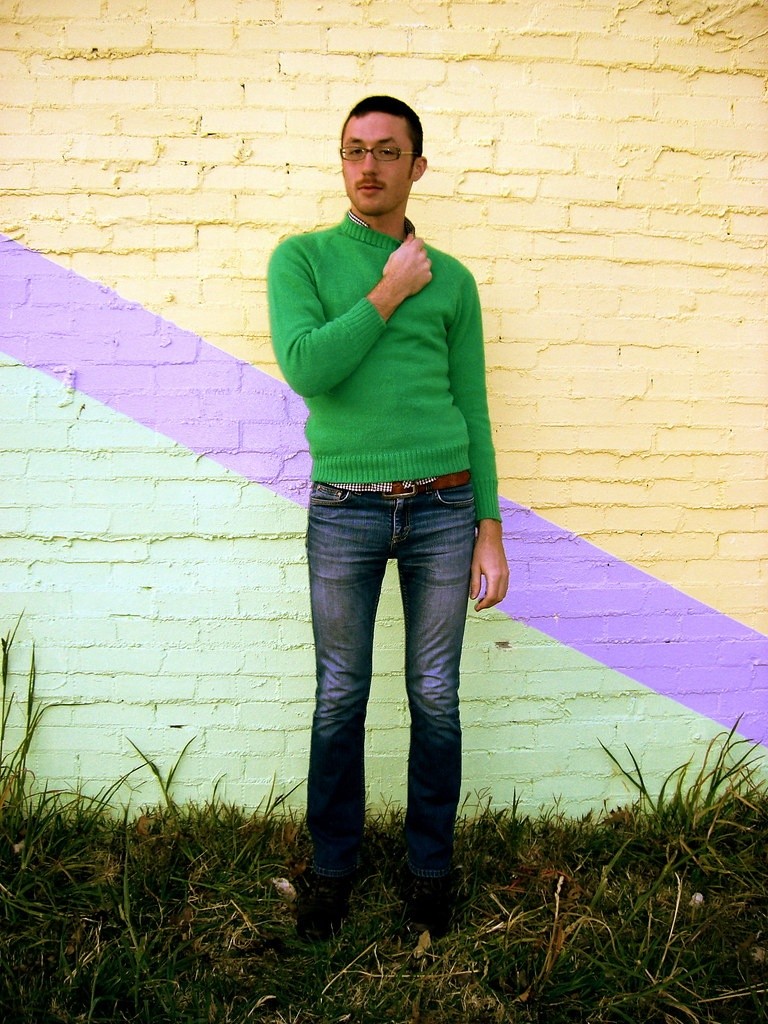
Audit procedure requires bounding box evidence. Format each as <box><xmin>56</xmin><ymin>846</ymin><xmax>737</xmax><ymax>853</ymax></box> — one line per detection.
<box><xmin>268</xmin><ymin>97</ymin><xmax>509</xmax><ymax>945</ymax></box>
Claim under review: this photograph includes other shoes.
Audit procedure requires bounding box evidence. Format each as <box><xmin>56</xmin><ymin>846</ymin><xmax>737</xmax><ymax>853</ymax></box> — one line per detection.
<box><xmin>398</xmin><ymin>861</ymin><xmax>457</xmax><ymax>936</ymax></box>
<box><xmin>295</xmin><ymin>866</ymin><xmax>353</xmax><ymax>945</ymax></box>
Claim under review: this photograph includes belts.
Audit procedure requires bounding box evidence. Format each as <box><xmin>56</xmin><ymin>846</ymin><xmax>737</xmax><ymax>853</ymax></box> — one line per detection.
<box><xmin>382</xmin><ymin>470</ymin><xmax>472</xmax><ymax>499</ymax></box>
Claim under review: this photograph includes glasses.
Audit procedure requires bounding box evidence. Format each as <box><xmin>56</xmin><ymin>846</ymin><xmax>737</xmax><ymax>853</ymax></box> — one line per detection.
<box><xmin>340</xmin><ymin>146</ymin><xmax>418</xmax><ymax>161</ymax></box>
